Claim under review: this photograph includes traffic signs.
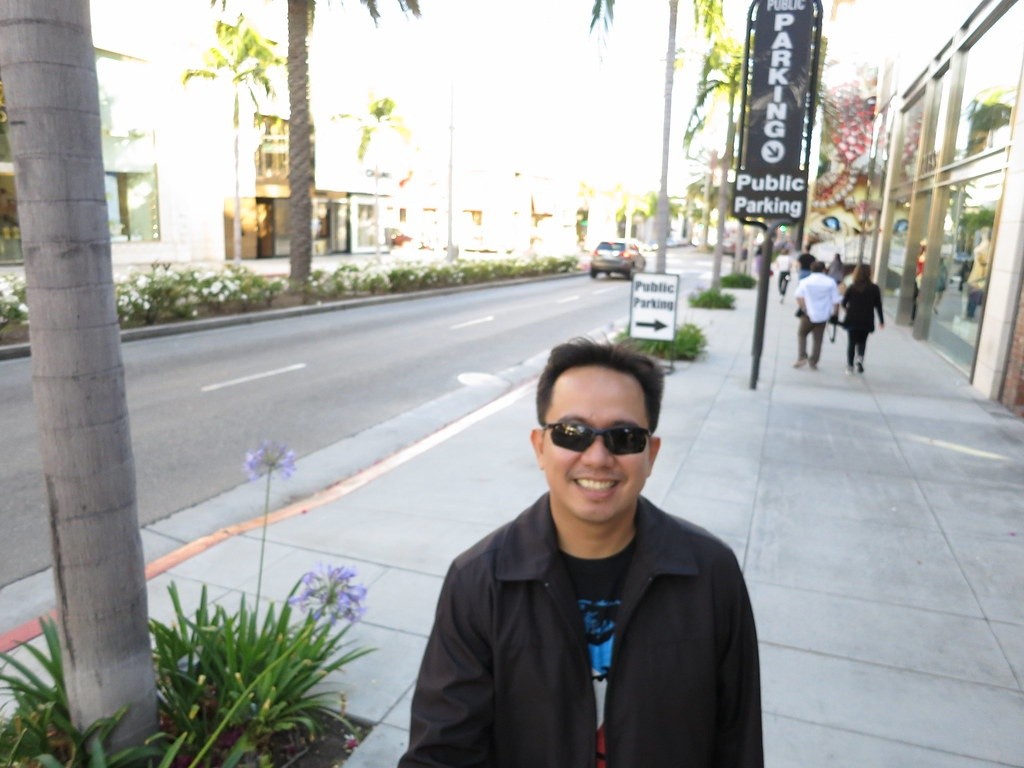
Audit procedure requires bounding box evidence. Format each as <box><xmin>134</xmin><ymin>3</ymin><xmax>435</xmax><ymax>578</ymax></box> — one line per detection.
<box><xmin>628</xmin><ymin>272</ymin><xmax>677</xmax><ymax>343</ymax></box>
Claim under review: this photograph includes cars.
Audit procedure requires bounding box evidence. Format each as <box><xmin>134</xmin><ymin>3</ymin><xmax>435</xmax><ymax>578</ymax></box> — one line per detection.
<box><xmin>589</xmin><ymin>240</ymin><xmax>644</xmax><ymax>281</ymax></box>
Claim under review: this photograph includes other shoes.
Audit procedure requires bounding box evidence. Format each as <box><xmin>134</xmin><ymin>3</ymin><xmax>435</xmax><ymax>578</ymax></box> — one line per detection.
<box><xmin>793</xmin><ymin>354</ymin><xmax>808</xmax><ymax>369</ymax></box>
<box><xmin>846</xmin><ymin>366</ymin><xmax>852</xmax><ymax>374</ymax></box>
<box><xmin>856</xmin><ymin>361</ymin><xmax>863</xmax><ymax>373</ymax></box>
<box><xmin>808</xmin><ymin>359</ymin><xmax>817</xmax><ymax>370</ymax></box>
<box><xmin>932</xmin><ymin>307</ymin><xmax>937</xmax><ymax>315</ymax></box>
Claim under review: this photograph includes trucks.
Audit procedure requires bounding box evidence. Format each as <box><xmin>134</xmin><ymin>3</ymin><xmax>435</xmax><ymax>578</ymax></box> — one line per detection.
<box><xmin>769</xmin><ymin>231</ymin><xmax>911</xmax><ymax>293</ymax></box>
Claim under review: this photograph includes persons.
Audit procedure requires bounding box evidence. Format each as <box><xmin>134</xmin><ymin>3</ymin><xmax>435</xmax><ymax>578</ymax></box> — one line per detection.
<box><xmin>934</xmin><ymin>257</ymin><xmax>949</xmax><ymax>315</ymax></box>
<box><xmin>827</xmin><ymin>253</ymin><xmax>846</xmax><ymax>324</ymax></box>
<box><xmin>754</xmin><ymin>245</ymin><xmax>774</xmax><ymax>277</ymax></box>
<box><xmin>395</xmin><ymin>333</ymin><xmax>769</xmax><ymax>768</ymax></box>
<box><xmin>776</xmin><ymin>247</ymin><xmax>791</xmax><ymax>304</ymax></box>
<box><xmin>841</xmin><ymin>263</ymin><xmax>885</xmax><ymax>377</ymax></box>
<box><xmin>958</xmin><ymin>258</ymin><xmax>975</xmax><ymax>291</ymax></box>
<box><xmin>795</xmin><ymin>243</ymin><xmax>815</xmax><ymax>275</ymax></box>
<box><xmin>794</xmin><ymin>259</ymin><xmax>840</xmax><ymax>371</ymax></box>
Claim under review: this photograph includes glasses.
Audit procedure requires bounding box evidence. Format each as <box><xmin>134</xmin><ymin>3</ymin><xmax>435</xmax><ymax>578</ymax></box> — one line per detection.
<box><xmin>543</xmin><ymin>422</ymin><xmax>651</xmax><ymax>454</ymax></box>
